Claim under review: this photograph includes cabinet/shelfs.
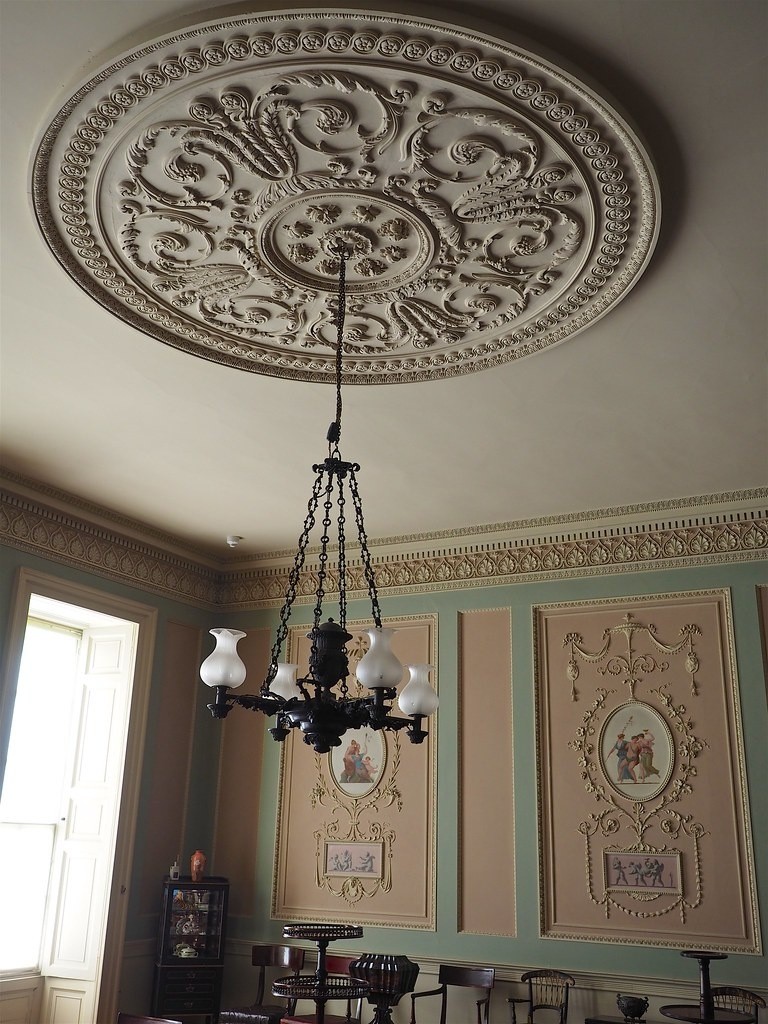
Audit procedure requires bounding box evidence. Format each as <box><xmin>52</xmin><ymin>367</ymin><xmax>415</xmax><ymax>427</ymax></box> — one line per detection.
<box><xmin>154</xmin><ymin>875</ymin><xmax>229</xmax><ymax>1023</ymax></box>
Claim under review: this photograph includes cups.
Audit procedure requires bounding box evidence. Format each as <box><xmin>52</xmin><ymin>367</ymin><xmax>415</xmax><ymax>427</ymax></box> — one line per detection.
<box><xmin>178</xmin><ymin>946</ymin><xmax>198</xmax><ymax>956</ymax></box>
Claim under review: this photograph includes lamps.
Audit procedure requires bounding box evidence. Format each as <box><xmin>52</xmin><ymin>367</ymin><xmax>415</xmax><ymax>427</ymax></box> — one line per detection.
<box><xmin>199</xmin><ymin>265</ymin><xmax>439</xmax><ymax>753</ymax></box>
<box><xmin>348</xmin><ymin>953</ymin><xmax>420</xmax><ymax>1024</ymax></box>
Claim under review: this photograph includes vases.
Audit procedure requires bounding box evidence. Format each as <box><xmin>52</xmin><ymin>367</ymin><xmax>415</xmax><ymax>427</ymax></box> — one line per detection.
<box><xmin>190</xmin><ymin>849</ymin><xmax>207</xmax><ymax>882</ymax></box>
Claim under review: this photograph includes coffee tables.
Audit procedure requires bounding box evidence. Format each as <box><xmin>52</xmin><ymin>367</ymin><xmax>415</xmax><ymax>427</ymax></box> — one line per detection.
<box><xmin>585</xmin><ymin>1014</ymin><xmax>647</xmax><ymax>1024</ymax></box>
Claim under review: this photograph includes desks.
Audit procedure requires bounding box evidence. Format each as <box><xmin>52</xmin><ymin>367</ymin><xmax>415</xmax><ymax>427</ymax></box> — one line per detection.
<box><xmin>272</xmin><ymin>923</ymin><xmax>371</xmax><ymax>1024</ymax></box>
<box><xmin>659</xmin><ymin>950</ymin><xmax>755</xmax><ymax>1024</ymax></box>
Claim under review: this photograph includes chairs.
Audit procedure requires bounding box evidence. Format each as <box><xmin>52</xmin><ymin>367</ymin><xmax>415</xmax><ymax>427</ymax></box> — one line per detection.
<box><xmin>280</xmin><ymin>955</ymin><xmax>363</xmax><ymax>1024</ymax></box>
<box><xmin>408</xmin><ymin>964</ymin><xmax>495</xmax><ymax>1024</ymax></box>
<box><xmin>506</xmin><ymin>969</ymin><xmax>576</xmax><ymax>1024</ymax></box>
<box><xmin>709</xmin><ymin>987</ymin><xmax>767</xmax><ymax>1024</ymax></box>
<box><xmin>217</xmin><ymin>944</ymin><xmax>304</xmax><ymax>1024</ymax></box>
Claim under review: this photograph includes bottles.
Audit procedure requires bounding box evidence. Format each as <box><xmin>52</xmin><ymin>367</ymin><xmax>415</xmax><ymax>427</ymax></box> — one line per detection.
<box><xmin>170</xmin><ymin>862</ymin><xmax>180</xmax><ymax>880</ymax></box>
<box><xmin>191</xmin><ymin>849</ymin><xmax>206</xmax><ymax>881</ymax></box>
